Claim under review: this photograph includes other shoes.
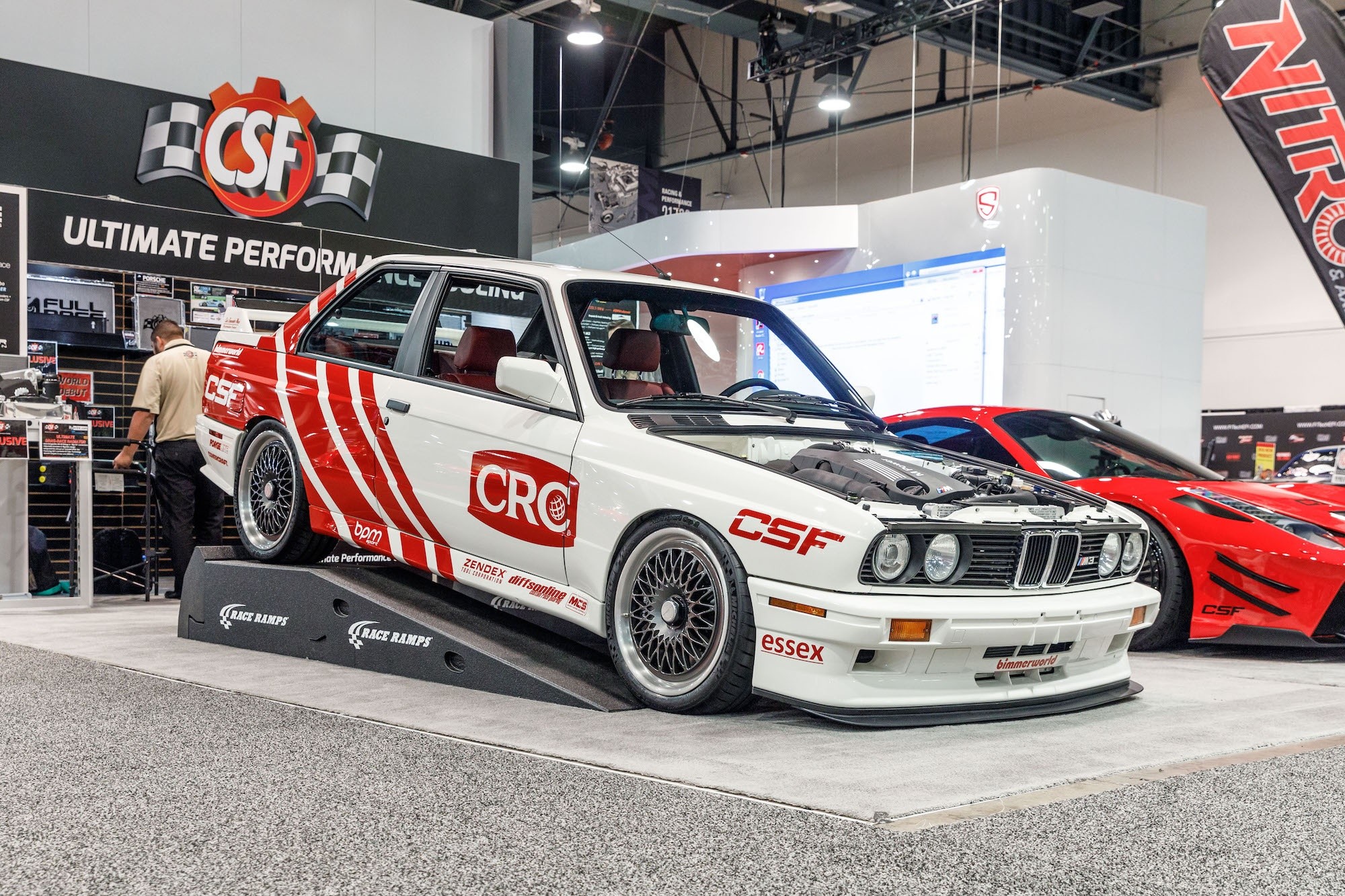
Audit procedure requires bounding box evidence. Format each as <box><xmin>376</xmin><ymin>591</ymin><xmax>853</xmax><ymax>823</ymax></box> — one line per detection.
<box><xmin>165</xmin><ymin>591</ymin><xmax>181</xmax><ymax>599</ymax></box>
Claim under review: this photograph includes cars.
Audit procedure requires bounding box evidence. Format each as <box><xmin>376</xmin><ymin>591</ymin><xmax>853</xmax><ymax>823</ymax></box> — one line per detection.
<box><xmin>1269</xmin><ymin>443</ymin><xmax>1345</xmax><ymax>485</ymax></box>
<box><xmin>881</xmin><ymin>405</ymin><xmax>1344</xmax><ymax>654</ymax></box>
<box><xmin>196</xmin><ymin>250</ymin><xmax>1165</xmax><ymax>728</ymax></box>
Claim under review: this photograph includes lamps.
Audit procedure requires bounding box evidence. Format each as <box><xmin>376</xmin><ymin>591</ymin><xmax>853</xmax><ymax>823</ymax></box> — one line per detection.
<box><xmin>560</xmin><ymin>137</ymin><xmax>587</xmax><ymax>172</ymax></box>
<box><xmin>567</xmin><ymin>0</ymin><xmax>604</xmax><ymax>46</ymax></box>
<box><xmin>818</xmin><ymin>84</ymin><xmax>850</xmax><ymax>111</ymax></box>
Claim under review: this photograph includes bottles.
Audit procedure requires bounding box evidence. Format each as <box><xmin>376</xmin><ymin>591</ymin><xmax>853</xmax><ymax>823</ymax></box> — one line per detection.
<box><xmin>41</xmin><ymin>373</ymin><xmax>62</xmax><ymax>401</ymax></box>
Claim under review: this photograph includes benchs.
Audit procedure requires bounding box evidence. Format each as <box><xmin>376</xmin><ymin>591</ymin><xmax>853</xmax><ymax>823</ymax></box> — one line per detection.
<box><xmin>310</xmin><ymin>335</ymin><xmax>458</xmax><ymax>376</ymax></box>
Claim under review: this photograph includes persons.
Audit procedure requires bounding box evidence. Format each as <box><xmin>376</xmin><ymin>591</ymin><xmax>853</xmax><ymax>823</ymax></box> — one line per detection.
<box><xmin>111</xmin><ymin>319</ymin><xmax>225</xmax><ymax>599</ymax></box>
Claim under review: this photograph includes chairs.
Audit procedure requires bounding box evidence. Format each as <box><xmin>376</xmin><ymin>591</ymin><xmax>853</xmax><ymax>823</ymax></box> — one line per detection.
<box><xmin>441</xmin><ymin>326</ymin><xmax>517</xmax><ymax>394</ymax></box>
<box><xmin>598</xmin><ymin>329</ymin><xmax>675</xmax><ymax>399</ymax></box>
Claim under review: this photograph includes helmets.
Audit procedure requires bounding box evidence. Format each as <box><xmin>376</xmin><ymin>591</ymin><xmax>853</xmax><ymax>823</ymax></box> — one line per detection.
<box><xmin>1092</xmin><ymin>409</ymin><xmax>1122</xmax><ymax>428</ymax></box>
